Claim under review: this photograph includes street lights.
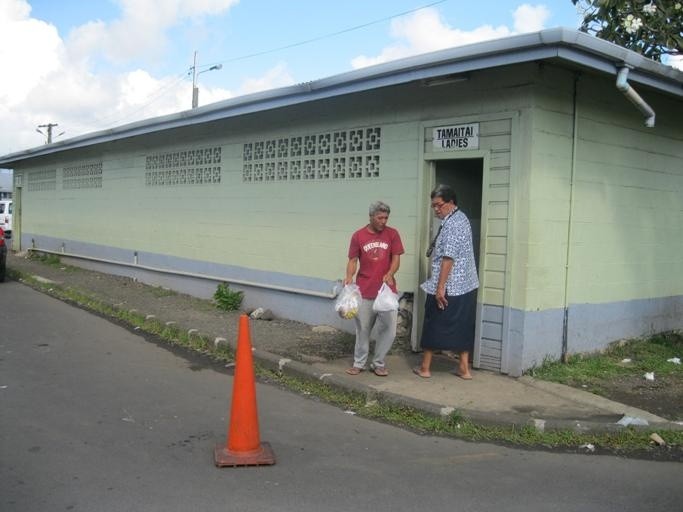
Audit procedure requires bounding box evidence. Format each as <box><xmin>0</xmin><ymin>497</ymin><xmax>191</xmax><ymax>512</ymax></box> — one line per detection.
<box><xmin>34</xmin><ymin>127</ymin><xmax>66</xmax><ymax>146</ymax></box>
<box><xmin>191</xmin><ymin>64</ymin><xmax>221</xmax><ymax>109</ymax></box>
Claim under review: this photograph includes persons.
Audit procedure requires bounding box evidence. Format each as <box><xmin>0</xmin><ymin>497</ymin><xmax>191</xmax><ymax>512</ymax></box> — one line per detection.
<box><xmin>410</xmin><ymin>182</ymin><xmax>479</xmax><ymax>380</ymax></box>
<box><xmin>341</xmin><ymin>200</ymin><xmax>404</xmax><ymax>377</ymax></box>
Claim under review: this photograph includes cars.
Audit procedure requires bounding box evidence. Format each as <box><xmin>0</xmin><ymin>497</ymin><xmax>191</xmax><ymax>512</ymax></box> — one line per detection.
<box><xmin>0</xmin><ymin>226</ymin><xmax>7</xmax><ymax>283</ymax></box>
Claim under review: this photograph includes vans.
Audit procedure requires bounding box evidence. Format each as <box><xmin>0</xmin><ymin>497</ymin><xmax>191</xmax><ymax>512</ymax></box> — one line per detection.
<box><xmin>0</xmin><ymin>198</ymin><xmax>13</xmax><ymax>238</ymax></box>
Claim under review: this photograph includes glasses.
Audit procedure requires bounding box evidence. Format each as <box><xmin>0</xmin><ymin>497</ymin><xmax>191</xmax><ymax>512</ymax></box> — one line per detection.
<box><xmin>431</xmin><ymin>202</ymin><xmax>447</xmax><ymax>208</ymax></box>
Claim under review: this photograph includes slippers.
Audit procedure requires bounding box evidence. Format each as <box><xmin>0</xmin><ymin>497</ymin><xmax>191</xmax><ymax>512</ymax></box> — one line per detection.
<box><xmin>449</xmin><ymin>368</ymin><xmax>472</xmax><ymax>380</ymax></box>
<box><xmin>374</xmin><ymin>367</ymin><xmax>388</xmax><ymax>376</ymax></box>
<box><xmin>345</xmin><ymin>367</ymin><xmax>361</xmax><ymax>375</ymax></box>
<box><xmin>413</xmin><ymin>366</ymin><xmax>431</xmax><ymax>378</ymax></box>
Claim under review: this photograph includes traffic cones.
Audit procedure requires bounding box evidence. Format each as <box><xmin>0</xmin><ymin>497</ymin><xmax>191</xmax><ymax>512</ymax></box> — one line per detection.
<box><xmin>212</xmin><ymin>311</ymin><xmax>277</xmax><ymax>468</ymax></box>
